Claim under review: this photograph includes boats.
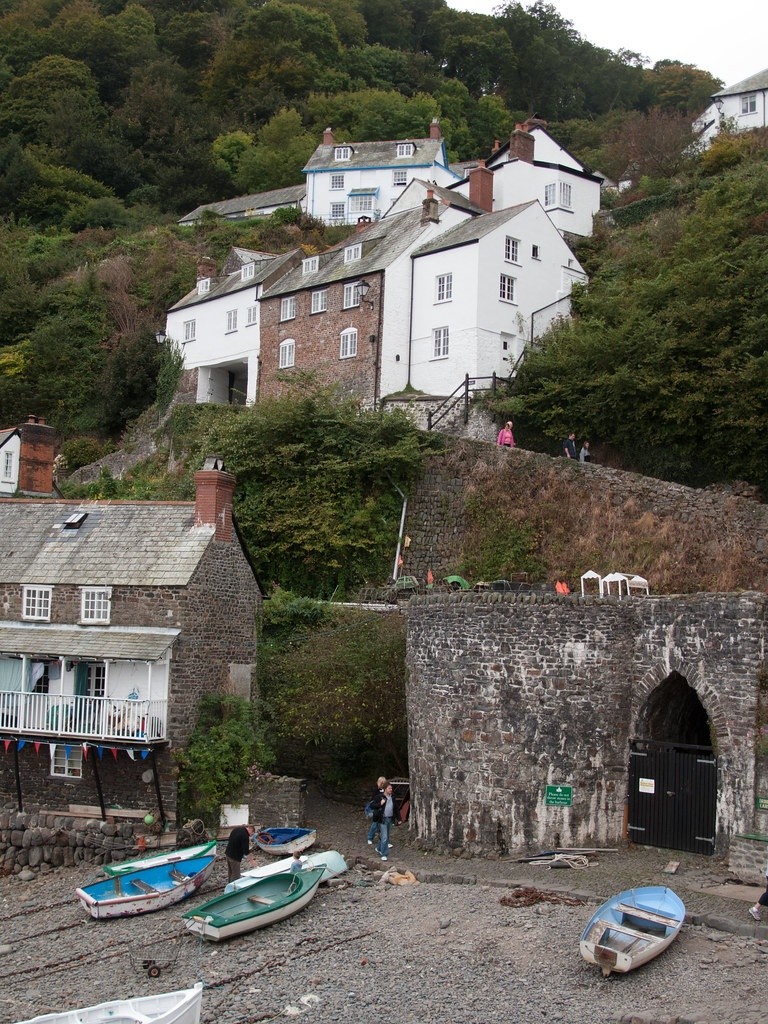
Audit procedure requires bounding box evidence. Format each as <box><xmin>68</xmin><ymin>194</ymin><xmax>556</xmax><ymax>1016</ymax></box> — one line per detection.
<box><xmin>100</xmin><ymin>839</ymin><xmax>215</xmax><ymax>882</ymax></box>
<box><xmin>180</xmin><ymin>863</ymin><xmax>328</xmax><ymax>937</ymax></box>
<box><xmin>224</xmin><ymin>850</ymin><xmax>348</xmax><ymax>895</ymax></box>
<box><xmin>74</xmin><ymin>852</ymin><xmax>218</xmax><ymax>919</ymax></box>
<box><xmin>252</xmin><ymin>826</ymin><xmax>317</xmax><ymax>856</ymax></box>
<box><xmin>579</xmin><ymin>885</ymin><xmax>687</xmax><ymax>977</ymax></box>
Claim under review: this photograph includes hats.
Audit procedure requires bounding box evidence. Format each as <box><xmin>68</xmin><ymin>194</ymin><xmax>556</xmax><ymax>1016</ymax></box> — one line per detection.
<box><xmin>507</xmin><ymin>421</ymin><xmax>513</xmax><ymax>429</ymax></box>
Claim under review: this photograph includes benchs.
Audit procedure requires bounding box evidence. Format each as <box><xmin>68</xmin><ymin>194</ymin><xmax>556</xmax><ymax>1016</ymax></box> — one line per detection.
<box><xmin>598</xmin><ymin>918</ymin><xmax>665</xmax><ymax>943</ymax></box>
<box><xmin>584</xmin><ymin>922</ymin><xmax>610</xmax><ymax>945</ymax></box>
<box><xmin>131</xmin><ymin>879</ymin><xmax>159</xmax><ymax>894</ymax></box>
<box><xmin>616</xmin><ymin>903</ymin><xmax>680</xmax><ymax>928</ymax></box>
<box><xmin>170</xmin><ymin>870</ymin><xmax>189</xmax><ymax>882</ymax></box>
<box><xmin>626</xmin><ymin>938</ymin><xmax>655</xmax><ymax>958</ymax></box>
<box><xmin>247</xmin><ymin>895</ymin><xmax>276</xmax><ymax>905</ymax></box>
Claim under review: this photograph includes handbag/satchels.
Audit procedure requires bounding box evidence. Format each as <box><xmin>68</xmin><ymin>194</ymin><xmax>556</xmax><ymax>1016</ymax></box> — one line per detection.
<box><xmin>364</xmin><ymin>800</ymin><xmax>375</xmax><ymax>819</ymax></box>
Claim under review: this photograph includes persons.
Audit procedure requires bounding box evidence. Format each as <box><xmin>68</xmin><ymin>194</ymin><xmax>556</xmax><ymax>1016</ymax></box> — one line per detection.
<box><xmin>367</xmin><ymin>777</ymin><xmax>395</xmax><ymax>848</ymax></box>
<box><xmin>291</xmin><ymin>851</ymin><xmax>302</xmax><ymax>871</ymax></box>
<box><xmin>580</xmin><ymin>441</ymin><xmax>594</xmax><ymax>466</ymax></box>
<box><xmin>562</xmin><ymin>433</ymin><xmax>576</xmax><ymax>460</ymax></box>
<box><xmin>496</xmin><ymin>422</ymin><xmax>516</xmax><ymax>448</ymax></box>
<box><xmin>368</xmin><ymin>783</ymin><xmax>402</xmax><ymax>860</ymax></box>
<box><xmin>749</xmin><ymin>869</ymin><xmax>768</xmax><ymax>920</ymax></box>
<box><xmin>224</xmin><ymin>823</ymin><xmax>255</xmax><ymax>884</ymax></box>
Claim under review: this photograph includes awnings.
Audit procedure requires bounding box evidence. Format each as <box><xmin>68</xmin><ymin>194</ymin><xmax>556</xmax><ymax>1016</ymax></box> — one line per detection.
<box><xmin>0</xmin><ymin>627</ymin><xmax>181</xmax><ymax>662</ymax></box>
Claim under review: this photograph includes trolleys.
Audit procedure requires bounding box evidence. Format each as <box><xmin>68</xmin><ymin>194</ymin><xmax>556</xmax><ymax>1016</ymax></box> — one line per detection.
<box><xmin>125</xmin><ymin>917</ymin><xmax>197</xmax><ymax>978</ymax></box>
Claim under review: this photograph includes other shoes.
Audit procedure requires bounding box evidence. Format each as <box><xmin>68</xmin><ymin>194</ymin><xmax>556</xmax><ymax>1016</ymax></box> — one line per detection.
<box><xmin>368</xmin><ymin>839</ymin><xmax>372</xmax><ymax>845</ymax></box>
<box><xmin>381</xmin><ymin>856</ymin><xmax>387</xmax><ymax>861</ymax></box>
<box><xmin>387</xmin><ymin>844</ymin><xmax>393</xmax><ymax>848</ymax></box>
<box><xmin>374</xmin><ymin>848</ymin><xmax>383</xmax><ymax>855</ymax></box>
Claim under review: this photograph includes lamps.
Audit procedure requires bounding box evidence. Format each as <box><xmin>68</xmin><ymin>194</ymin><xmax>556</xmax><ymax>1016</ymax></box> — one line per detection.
<box><xmin>66</xmin><ymin>661</ymin><xmax>75</xmax><ymax>672</ymax></box>
<box><xmin>355</xmin><ymin>279</ymin><xmax>374</xmax><ymax>311</ymax></box>
